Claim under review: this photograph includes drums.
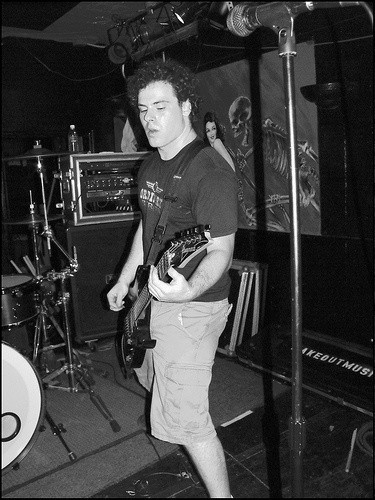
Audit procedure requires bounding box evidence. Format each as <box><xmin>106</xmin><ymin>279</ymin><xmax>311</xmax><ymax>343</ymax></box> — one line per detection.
<box><xmin>1</xmin><ymin>340</ymin><xmax>46</xmax><ymax>478</ymax></box>
<box><xmin>0</xmin><ymin>274</ymin><xmax>40</xmax><ymax>327</ymax></box>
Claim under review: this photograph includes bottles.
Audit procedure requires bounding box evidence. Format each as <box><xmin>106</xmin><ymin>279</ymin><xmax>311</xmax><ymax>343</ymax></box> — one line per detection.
<box><xmin>68</xmin><ymin>125</ymin><xmax>79</xmax><ymax>153</ymax></box>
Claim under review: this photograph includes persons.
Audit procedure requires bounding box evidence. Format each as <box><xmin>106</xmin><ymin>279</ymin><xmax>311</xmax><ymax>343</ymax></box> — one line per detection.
<box><xmin>105</xmin><ymin>58</ymin><xmax>239</xmax><ymax>499</ymax></box>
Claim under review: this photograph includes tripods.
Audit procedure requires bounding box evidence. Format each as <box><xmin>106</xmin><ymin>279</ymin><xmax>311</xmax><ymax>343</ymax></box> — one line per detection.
<box><xmin>28</xmin><ymin>222</ymin><xmax>122</xmax><ymax>435</ymax></box>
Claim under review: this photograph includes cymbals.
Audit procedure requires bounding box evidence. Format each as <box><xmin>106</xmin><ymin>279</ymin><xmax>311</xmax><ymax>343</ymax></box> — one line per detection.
<box><xmin>2</xmin><ymin>214</ymin><xmax>66</xmax><ymax>226</ymax></box>
<box><xmin>2</xmin><ymin>148</ymin><xmax>73</xmax><ymax>161</ymax></box>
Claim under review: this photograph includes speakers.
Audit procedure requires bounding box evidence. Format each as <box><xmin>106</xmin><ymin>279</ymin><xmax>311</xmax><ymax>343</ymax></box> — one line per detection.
<box><xmin>57</xmin><ymin>220</ymin><xmax>150</xmax><ymax>346</ymax></box>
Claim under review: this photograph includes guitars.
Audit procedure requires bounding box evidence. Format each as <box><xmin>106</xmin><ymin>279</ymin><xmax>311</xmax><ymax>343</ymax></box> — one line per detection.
<box><xmin>114</xmin><ymin>223</ymin><xmax>215</xmax><ymax>369</ymax></box>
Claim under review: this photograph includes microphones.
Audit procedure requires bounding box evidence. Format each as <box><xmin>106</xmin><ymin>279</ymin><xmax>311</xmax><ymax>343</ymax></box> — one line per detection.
<box><xmin>226</xmin><ymin>1</ymin><xmax>365</xmax><ymax>37</ymax></box>
